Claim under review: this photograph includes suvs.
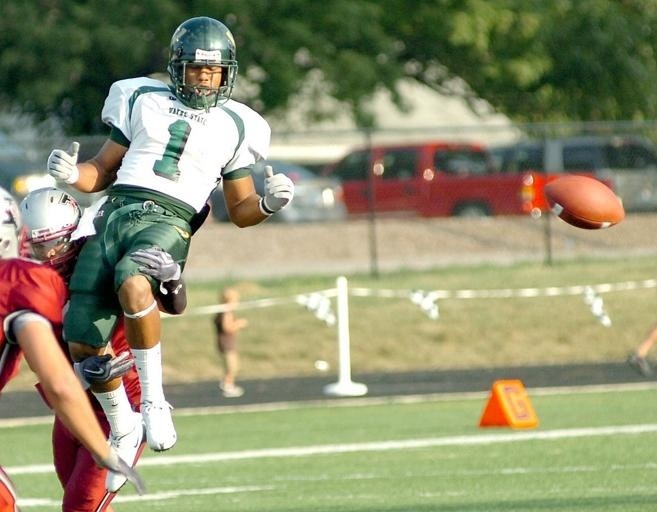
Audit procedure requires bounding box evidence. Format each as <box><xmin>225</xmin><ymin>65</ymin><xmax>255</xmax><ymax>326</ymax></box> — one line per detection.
<box><xmin>498</xmin><ymin>134</ymin><xmax>656</xmax><ymax>212</ymax></box>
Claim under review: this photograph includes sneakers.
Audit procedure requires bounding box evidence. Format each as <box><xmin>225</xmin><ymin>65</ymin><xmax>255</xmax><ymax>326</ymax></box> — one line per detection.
<box><xmin>105</xmin><ymin>412</ymin><xmax>145</xmax><ymax>493</ymax></box>
<box><xmin>218</xmin><ymin>379</ymin><xmax>244</xmax><ymax>397</ymax></box>
<box><xmin>140</xmin><ymin>394</ymin><xmax>177</xmax><ymax>451</ymax></box>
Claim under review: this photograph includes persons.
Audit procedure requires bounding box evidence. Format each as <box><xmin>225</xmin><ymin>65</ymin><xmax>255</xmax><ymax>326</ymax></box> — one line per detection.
<box><xmin>19</xmin><ymin>187</ymin><xmax>187</xmax><ymax>512</ymax></box>
<box><xmin>627</xmin><ymin>326</ymin><xmax>657</xmax><ymax>376</ymax></box>
<box><xmin>47</xmin><ymin>17</ymin><xmax>295</xmax><ymax>493</ymax></box>
<box><xmin>0</xmin><ymin>186</ymin><xmax>146</xmax><ymax>512</ymax></box>
<box><xmin>214</xmin><ymin>286</ymin><xmax>248</xmax><ymax>398</ymax></box>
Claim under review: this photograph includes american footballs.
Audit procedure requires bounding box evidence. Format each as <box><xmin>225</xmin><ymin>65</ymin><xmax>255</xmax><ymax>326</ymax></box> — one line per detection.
<box><xmin>544</xmin><ymin>176</ymin><xmax>625</xmax><ymax>228</ymax></box>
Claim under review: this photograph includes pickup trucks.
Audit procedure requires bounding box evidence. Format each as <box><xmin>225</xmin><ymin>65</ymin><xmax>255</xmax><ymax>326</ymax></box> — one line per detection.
<box><xmin>324</xmin><ymin>136</ymin><xmax>613</xmax><ymax>224</ymax></box>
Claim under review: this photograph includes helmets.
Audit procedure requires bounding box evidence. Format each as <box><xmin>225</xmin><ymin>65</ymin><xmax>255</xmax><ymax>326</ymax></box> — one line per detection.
<box><xmin>17</xmin><ymin>186</ymin><xmax>83</xmax><ymax>264</ymax></box>
<box><xmin>168</xmin><ymin>15</ymin><xmax>236</xmax><ymax>110</ymax></box>
<box><xmin>0</xmin><ymin>186</ymin><xmax>20</xmax><ymax>257</ymax></box>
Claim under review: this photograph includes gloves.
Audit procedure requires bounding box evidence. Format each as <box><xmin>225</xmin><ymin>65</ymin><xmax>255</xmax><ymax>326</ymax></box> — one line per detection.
<box><xmin>259</xmin><ymin>162</ymin><xmax>295</xmax><ymax>213</ymax></box>
<box><xmin>75</xmin><ymin>350</ymin><xmax>135</xmax><ymax>391</ymax></box>
<box><xmin>45</xmin><ymin>140</ymin><xmax>80</xmax><ymax>184</ymax></box>
<box><xmin>98</xmin><ymin>452</ymin><xmax>146</xmax><ymax>495</ymax></box>
<box><xmin>131</xmin><ymin>245</ymin><xmax>181</xmax><ymax>282</ymax></box>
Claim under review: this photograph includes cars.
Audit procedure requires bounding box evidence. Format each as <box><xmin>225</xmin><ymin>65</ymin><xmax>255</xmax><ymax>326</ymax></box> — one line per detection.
<box><xmin>206</xmin><ymin>157</ymin><xmax>349</xmax><ymax>223</ymax></box>
